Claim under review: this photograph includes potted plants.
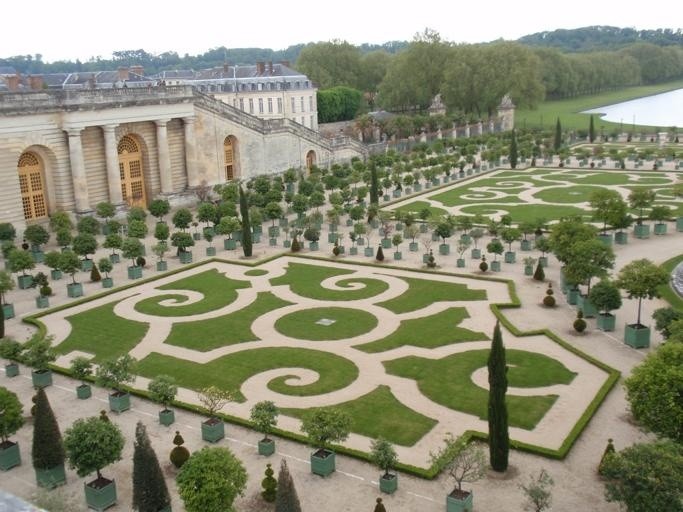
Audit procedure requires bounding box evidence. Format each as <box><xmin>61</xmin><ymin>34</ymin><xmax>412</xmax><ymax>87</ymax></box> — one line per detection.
<box><xmin>0</xmin><ymin>386</ymin><xmax>22</xmax><ymax>471</ymax></box>
<box><xmin>370</xmin><ymin>436</ymin><xmax>398</xmax><ymax>495</ymax></box>
<box><xmin>300</xmin><ymin>405</ymin><xmax>350</xmax><ymax>475</ymax></box>
<box><xmin>194</xmin><ymin>384</ymin><xmax>238</xmax><ymax>442</ymax></box>
<box><xmin>62</xmin><ymin>415</ymin><xmax>126</xmax><ymax>509</ymax></box>
<box><xmin>29</xmin><ymin>386</ymin><xmax>67</xmax><ymax>488</ymax></box>
<box><xmin>247</xmin><ymin>400</ymin><xmax>279</xmax><ymax>457</ymax></box>
<box><xmin>2</xmin><ymin>130</ymin><xmax>679</xmax><ymax>348</ymax></box>
<box><xmin>430</xmin><ymin>435</ymin><xmax>487</xmax><ymax>511</ymax></box>
<box><xmin>0</xmin><ymin>349</ymin><xmax>140</xmax><ymax>413</ymax></box>
<box><xmin>149</xmin><ymin>372</ymin><xmax>180</xmax><ymax>425</ymax></box>
